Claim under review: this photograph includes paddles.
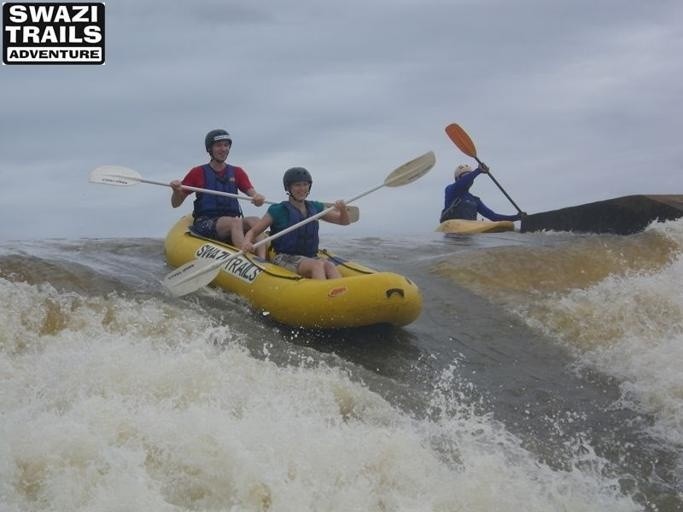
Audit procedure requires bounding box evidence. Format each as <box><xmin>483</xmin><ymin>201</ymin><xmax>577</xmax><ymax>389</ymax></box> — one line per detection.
<box><xmin>445</xmin><ymin>124</ymin><xmax>527</xmax><ymax>216</ymax></box>
<box><xmin>88</xmin><ymin>165</ymin><xmax>359</xmax><ymax>222</ymax></box>
<box><xmin>165</xmin><ymin>151</ymin><xmax>436</xmax><ymax>296</ymax></box>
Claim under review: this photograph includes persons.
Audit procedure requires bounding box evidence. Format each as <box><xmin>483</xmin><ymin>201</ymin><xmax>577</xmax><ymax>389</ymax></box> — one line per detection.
<box><xmin>440</xmin><ymin>161</ymin><xmax>527</xmax><ymax>222</ymax></box>
<box><xmin>170</xmin><ymin>130</ymin><xmax>266</xmax><ymax>261</ymax></box>
<box><xmin>242</xmin><ymin>168</ymin><xmax>351</xmax><ymax>279</ymax></box>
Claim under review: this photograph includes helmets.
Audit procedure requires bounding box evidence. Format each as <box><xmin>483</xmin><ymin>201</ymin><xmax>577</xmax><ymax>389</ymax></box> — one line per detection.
<box><xmin>206</xmin><ymin>130</ymin><xmax>232</xmax><ymax>151</ymax></box>
<box><xmin>455</xmin><ymin>165</ymin><xmax>473</xmax><ymax>181</ymax></box>
<box><xmin>283</xmin><ymin>167</ymin><xmax>311</xmax><ymax>191</ymax></box>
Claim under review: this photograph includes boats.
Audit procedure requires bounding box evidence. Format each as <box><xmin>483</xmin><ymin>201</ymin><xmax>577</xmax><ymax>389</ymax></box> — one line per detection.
<box><xmin>163</xmin><ymin>214</ymin><xmax>420</xmax><ymax>329</ymax></box>
<box><xmin>434</xmin><ymin>218</ymin><xmax>514</xmax><ymax>233</ymax></box>
